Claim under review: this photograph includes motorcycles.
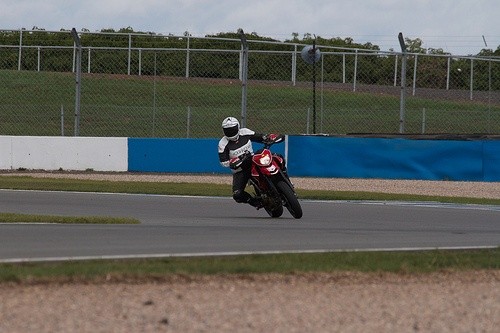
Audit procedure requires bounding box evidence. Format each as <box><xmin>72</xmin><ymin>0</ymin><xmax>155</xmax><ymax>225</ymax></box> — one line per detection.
<box><xmin>229</xmin><ymin>133</ymin><xmax>303</xmax><ymax>220</ymax></box>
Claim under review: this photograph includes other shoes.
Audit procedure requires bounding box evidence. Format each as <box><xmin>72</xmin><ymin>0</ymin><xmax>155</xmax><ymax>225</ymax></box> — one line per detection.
<box><xmin>283</xmin><ymin>170</ymin><xmax>295</xmax><ymax>188</ymax></box>
<box><xmin>244</xmin><ymin>194</ymin><xmax>262</xmax><ymax>209</ymax></box>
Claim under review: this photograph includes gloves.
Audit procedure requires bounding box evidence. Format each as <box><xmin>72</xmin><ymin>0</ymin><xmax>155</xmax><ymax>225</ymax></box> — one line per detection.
<box><xmin>267</xmin><ymin>133</ymin><xmax>277</xmax><ymax>141</ymax></box>
<box><xmin>230</xmin><ymin>156</ymin><xmax>243</xmax><ymax>170</ymax></box>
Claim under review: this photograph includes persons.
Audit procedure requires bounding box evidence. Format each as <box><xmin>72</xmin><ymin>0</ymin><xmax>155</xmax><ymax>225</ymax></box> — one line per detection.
<box><xmin>217</xmin><ymin>116</ymin><xmax>285</xmax><ymax>207</ymax></box>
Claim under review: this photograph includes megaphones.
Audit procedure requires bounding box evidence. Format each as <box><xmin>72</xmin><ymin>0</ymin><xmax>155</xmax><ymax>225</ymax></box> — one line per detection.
<box><xmin>301</xmin><ymin>44</ymin><xmax>321</xmax><ymax>64</ymax></box>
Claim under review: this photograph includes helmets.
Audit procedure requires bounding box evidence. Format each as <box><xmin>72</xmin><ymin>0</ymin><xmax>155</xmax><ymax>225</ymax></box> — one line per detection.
<box><xmin>221</xmin><ymin>117</ymin><xmax>241</xmax><ymax>141</ymax></box>
